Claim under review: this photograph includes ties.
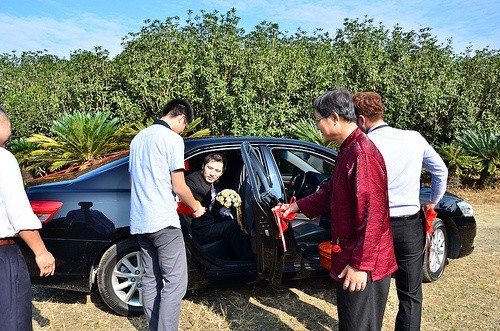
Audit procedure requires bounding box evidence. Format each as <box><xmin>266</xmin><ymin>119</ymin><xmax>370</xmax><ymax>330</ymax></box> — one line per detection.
<box><xmin>209</xmin><ymin>183</ymin><xmax>216</xmax><ymax>211</ymax></box>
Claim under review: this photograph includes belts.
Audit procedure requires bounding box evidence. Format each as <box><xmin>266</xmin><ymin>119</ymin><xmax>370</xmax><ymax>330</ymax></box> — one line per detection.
<box><xmin>389</xmin><ymin>213</ymin><xmax>419</xmax><ymax>221</ymax></box>
<box><xmin>0</xmin><ymin>240</ymin><xmax>15</xmax><ymax>245</ymax></box>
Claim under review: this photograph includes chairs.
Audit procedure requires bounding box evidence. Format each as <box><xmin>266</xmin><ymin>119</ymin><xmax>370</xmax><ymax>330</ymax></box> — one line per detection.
<box><xmin>292</xmin><ymin>223</ymin><xmax>328</xmax><ymax>255</ymax></box>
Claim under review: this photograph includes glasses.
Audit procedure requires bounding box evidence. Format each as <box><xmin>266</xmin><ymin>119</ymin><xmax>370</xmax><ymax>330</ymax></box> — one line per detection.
<box><xmin>315</xmin><ymin>115</ymin><xmax>325</xmax><ymax>123</ymax></box>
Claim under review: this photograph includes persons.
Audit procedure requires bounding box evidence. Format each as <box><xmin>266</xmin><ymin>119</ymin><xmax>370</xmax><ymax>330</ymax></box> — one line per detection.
<box><xmin>352</xmin><ymin>92</ymin><xmax>448</xmax><ymax>331</ymax></box>
<box><xmin>0</xmin><ymin>106</ymin><xmax>55</xmax><ymax>331</ymax></box>
<box><xmin>282</xmin><ymin>88</ymin><xmax>398</xmax><ymax>331</ymax></box>
<box><xmin>62</xmin><ymin>202</ymin><xmax>115</xmax><ymax>272</ymax></box>
<box><xmin>185</xmin><ymin>151</ymin><xmax>254</xmax><ymax>260</ymax></box>
<box><xmin>129</xmin><ymin>99</ymin><xmax>205</xmax><ymax>331</ymax></box>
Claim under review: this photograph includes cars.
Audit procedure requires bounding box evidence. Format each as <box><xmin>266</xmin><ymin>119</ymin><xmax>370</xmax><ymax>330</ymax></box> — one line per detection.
<box><xmin>16</xmin><ymin>136</ymin><xmax>477</xmax><ymax>319</ymax></box>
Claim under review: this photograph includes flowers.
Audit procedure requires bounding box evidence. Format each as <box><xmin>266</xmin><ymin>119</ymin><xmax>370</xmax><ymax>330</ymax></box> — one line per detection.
<box><xmin>214</xmin><ymin>189</ymin><xmax>243</xmax><ymax>220</ymax></box>
<box><xmin>331</xmin><ymin>237</ymin><xmax>343</xmax><ymax>256</ymax></box>
